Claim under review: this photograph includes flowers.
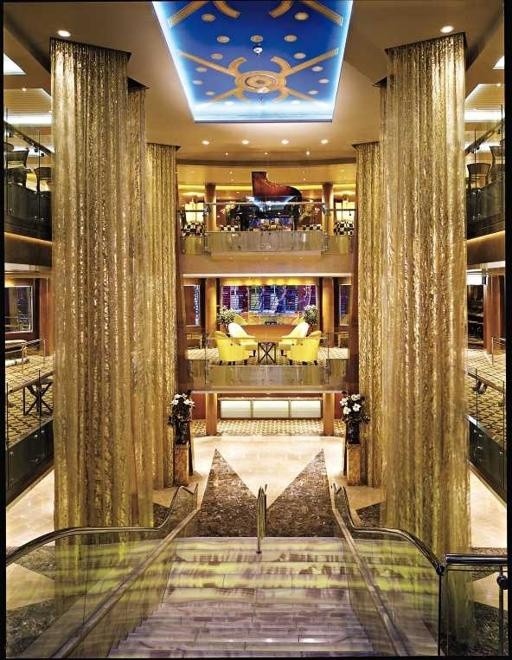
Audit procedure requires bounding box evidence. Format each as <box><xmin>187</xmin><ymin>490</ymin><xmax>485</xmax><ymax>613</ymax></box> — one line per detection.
<box><xmin>339</xmin><ymin>393</ymin><xmax>370</xmax><ymax>444</ymax></box>
<box><xmin>167</xmin><ymin>393</ymin><xmax>195</xmax><ymax>445</ymax></box>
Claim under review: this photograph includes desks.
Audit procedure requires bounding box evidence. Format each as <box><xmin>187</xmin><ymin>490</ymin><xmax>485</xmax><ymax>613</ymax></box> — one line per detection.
<box><xmin>258</xmin><ymin>341</ymin><xmax>277</xmax><ymax>364</ymax></box>
<box><xmin>22</xmin><ymin>379</ymin><xmax>53</xmax><ymax>415</ymax></box>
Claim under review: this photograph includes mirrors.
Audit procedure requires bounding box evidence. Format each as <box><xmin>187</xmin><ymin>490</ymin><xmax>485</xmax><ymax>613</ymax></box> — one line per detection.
<box><xmin>5</xmin><ymin>286</ymin><xmax>34</xmax><ymax>334</ymax></box>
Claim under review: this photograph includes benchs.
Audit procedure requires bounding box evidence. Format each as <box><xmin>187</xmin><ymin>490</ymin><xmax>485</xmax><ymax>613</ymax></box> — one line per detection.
<box><xmin>4</xmin><ymin>340</ymin><xmax>27</xmax><ymax>357</ymax></box>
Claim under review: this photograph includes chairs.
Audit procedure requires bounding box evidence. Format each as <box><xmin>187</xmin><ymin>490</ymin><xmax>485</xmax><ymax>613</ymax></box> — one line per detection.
<box><xmin>287</xmin><ymin>330</ymin><xmax>322</xmax><ymax>366</ymax></box>
<box><xmin>7</xmin><ymin>150</ymin><xmax>29</xmax><ymax>187</ymax></box>
<box><xmin>214</xmin><ymin>331</ymin><xmax>249</xmax><ymax>365</ymax></box>
<box><xmin>34</xmin><ymin>167</ymin><xmax>51</xmax><ymax>192</ymax></box>
<box><xmin>228</xmin><ymin>323</ymin><xmax>258</xmax><ymax>357</ymax></box>
<box><xmin>467</xmin><ymin>163</ymin><xmax>491</xmax><ymax>193</ymax></box>
<box><xmin>490</xmin><ymin>145</ymin><xmax>506</xmax><ymax>182</ymax></box>
<box><xmin>279</xmin><ymin>322</ymin><xmax>310</xmax><ymax>356</ymax></box>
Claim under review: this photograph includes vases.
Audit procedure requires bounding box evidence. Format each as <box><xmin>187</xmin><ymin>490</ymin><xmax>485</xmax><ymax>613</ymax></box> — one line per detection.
<box><xmin>346</xmin><ymin>441</ymin><xmax>362</xmax><ymax>486</ymax></box>
<box><xmin>174</xmin><ymin>441</ymin><xmax>189</xmax><ymax>485</ymax></box>
